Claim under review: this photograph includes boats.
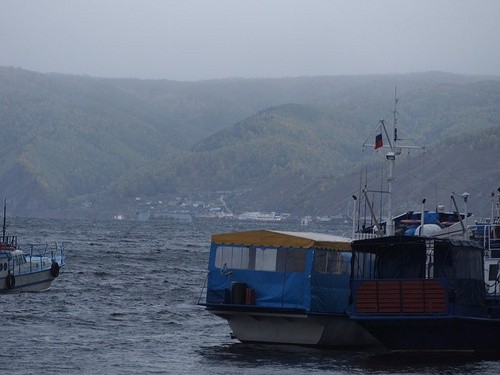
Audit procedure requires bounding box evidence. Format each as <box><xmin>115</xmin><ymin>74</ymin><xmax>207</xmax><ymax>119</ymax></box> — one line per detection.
<box><xmin>219</xmin><ymin>208</ymin><xmax>387</xmax><ymax>226</ymax></box>
<box><xmin>0</xmin><ymin>195</ymin><xmax>65</xmax><ymax>291</ymax></box>
<box><xmin>204</xmin><ymin>85</ymin><xmax>500</xmax><ymax>349</ymax></box>
